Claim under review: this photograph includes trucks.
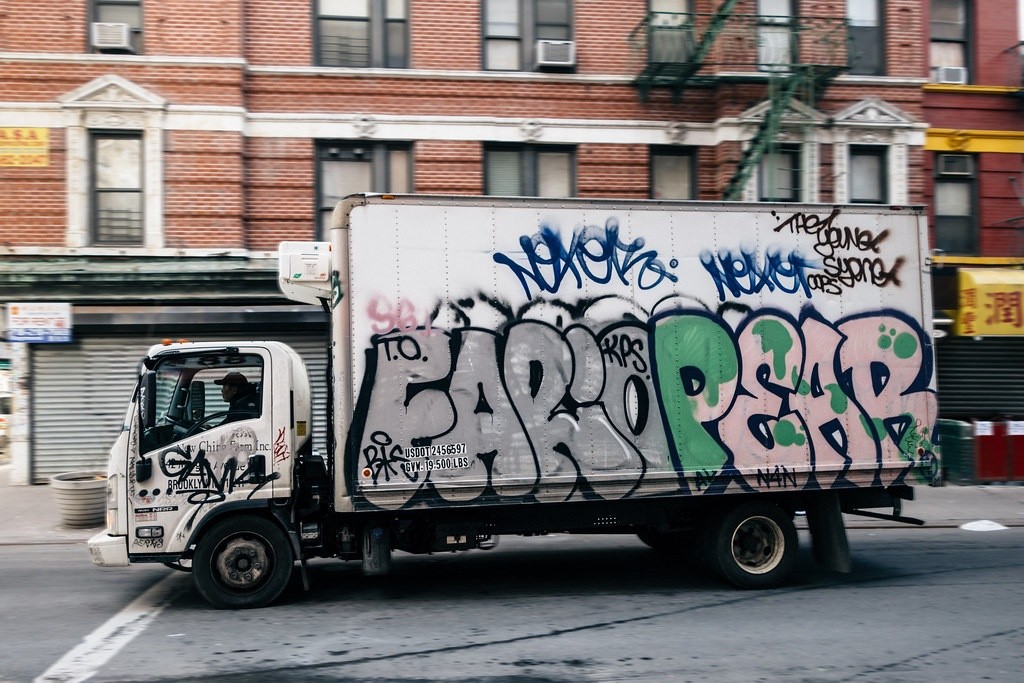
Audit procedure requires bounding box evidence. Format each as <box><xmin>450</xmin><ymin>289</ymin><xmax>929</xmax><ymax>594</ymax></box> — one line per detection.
<box><xmin>86</xmin><ymin>192</ymin><xmax>958</xmax><ymax>597</ymax></box>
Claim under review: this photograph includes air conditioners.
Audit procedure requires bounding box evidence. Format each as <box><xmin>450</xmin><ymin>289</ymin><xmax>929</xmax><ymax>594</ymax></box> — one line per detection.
<box><xmin>939</xmin><ymin>67</ymin><xmax>968</xmax><ymax>85</ymax></box>
<box><xmin>90</xmin><ymin>21</ymin><xmax>132</xmax><ymax>54</ymax></box>
<box><xmin>534</xmin><ymin>39</ymin><xmax>576</xmax><ymax>70</ymax></box>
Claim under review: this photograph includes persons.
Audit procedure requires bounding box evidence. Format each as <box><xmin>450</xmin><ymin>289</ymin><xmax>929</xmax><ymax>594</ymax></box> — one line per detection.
<box><xmin>214</xmin><ymin>372</ymin><xmax>259</xmax><ymax>426</ymax></box>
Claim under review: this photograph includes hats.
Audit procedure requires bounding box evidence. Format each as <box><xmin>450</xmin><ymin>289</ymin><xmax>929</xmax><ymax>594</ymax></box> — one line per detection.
<box><xmin>214</xmin><ymin>372</ymin><xmax>248</xmax><ymax>387</ymax></box>
